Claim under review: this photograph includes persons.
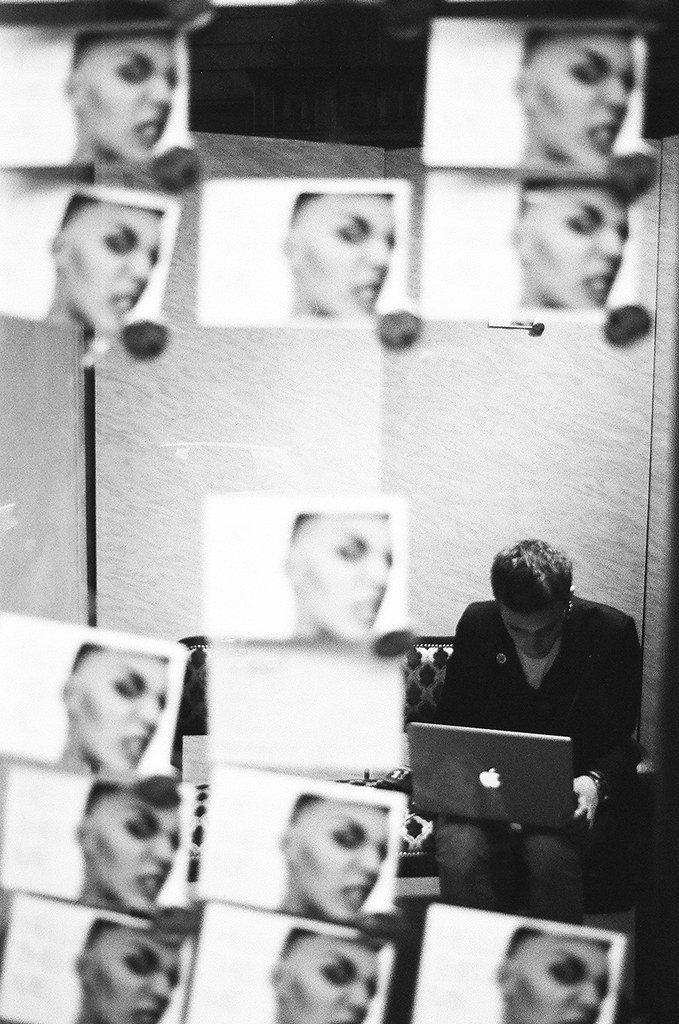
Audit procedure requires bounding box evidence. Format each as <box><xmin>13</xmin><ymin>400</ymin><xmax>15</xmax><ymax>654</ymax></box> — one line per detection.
<box><xmin>511</xmin><ymin>24</ymin><xmax>636</xmax><ymax>171</ymax></box>
<box><xmin>40</xmin><ymin>196</ymin><xmax>163</xmax><ymax>329</ymax></box>
<box><xmin>61</xmin><ymin>25</ymin><xmax>180</xmax><ymax>165</ymax></box>
<box><xmin>288</xmin><ymin>512</ymin><xmax>392</xmax><ymax>643</ymax></box>
<box><xmin>73</xmin><ymin>919</ymin><xmax>180</xmax><ymax>1024</ymax></box>
<box><xmin>435</xmin><ymin>539</ymin><xmax>644</xmax><ymax>924</ymax></box>
<box><xmin>59</xmin><ymin>643</ymin><xmax>169</xmax><ymax>776</ymax></box>
<box><xmin>75</xmin><ymin>776</ymin><xmax>181</xmax><ymax>914</ymax></box>
<box><xmin>282</xmin><ymin>193</ymin><xmax>396</xmax><ymax>319</ymax></box>
<box><xmin>278</xmin><ymin>794</ymin><xmax>389</xmax><ymax>924</ymax></box>
<box><xmin>268</xmin><ymin>929</ymin><xmax>380</xmax><ymax>1024</ymax></box>
<box><xmin>515</xmin><ymin>182</ymin><xmax>630</xmax><ymax>316</ymax></box>
<box><xmin>500</xmin><ymin>929</ymin><xmax>611</xmax><ymax>1024</ymax></box>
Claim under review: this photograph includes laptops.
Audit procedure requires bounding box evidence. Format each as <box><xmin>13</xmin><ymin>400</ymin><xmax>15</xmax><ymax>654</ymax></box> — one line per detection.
<box><xmin>405</xmin><ymin>722</ymin><xmax>581</xmax><ymax>831</ymax></box>
